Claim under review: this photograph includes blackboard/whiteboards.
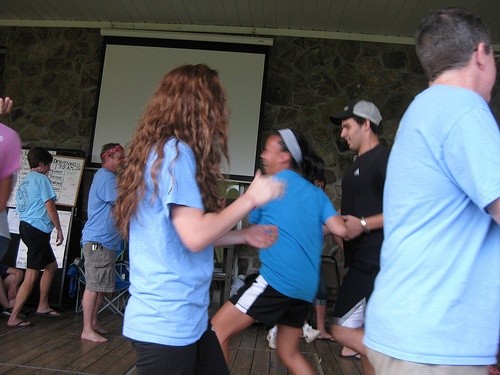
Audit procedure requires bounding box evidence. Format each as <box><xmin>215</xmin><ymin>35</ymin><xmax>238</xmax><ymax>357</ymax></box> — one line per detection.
<box><xmin>7</xmin><ymin>147</ymin><xmax>85</xmax><ymax>308</ymax></box>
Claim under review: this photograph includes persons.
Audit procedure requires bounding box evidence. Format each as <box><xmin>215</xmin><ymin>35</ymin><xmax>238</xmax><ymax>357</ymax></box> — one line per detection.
<box><xmin>110</xmin><ymin>62</ymin><xmax>285</xmax><ymax>375</ymax></box>
<box><xmin>7</xmin><ymin>147</ymin><xmax>62</xmax><ymax>326</ymax></box>
<box><xmin>311</xmin><ymin>177</ymin><xmax>341</xmax><ymax>341</ymax></box>
<box><xmin>265</xmin><ymin>319</ymin><xmax>321</xmax><ymax>349</ymax></box>
<box><xmin>210</xmin><ymin>128</ymin><xmax>348</xmax><ymax>375</ymax></box>
<box><xmin>341</xmin><ymin>346</ymin><xmax>362</xmax><ymax>359</ymax></box>
<box><xmin>79</xmin><ymin>142</ymin><xmax>125</xmax><ymax>343</ymax></box>
<box><xmin>0</xmin><ymin>262</ymin><xmax>24</xmax><ymax>316</ymax></box>
<box><xmin>362</xmin><ymin>8</ymin><xmax>499</xmax><ymax>375</ymax></box>
<box><xmin>0</xmin><ymin>98</ymin><xmax>23</xmax><ymax>211</ymax></box>
<box><xmin>329</xmin><ymin>99</ymin><xmax>391</xmax><ymax>374</ymax></box>
<box><xmin>0</xmin><ymin>213</ymin><xmax>10</xmax><ymax>264</ymax></box>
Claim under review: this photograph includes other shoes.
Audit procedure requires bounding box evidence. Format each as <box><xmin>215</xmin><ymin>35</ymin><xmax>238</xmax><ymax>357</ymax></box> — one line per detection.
<box><xmin>2</xmin><ymin>307</ymin><xmax>26</xmax><ymax>318</ymax></box>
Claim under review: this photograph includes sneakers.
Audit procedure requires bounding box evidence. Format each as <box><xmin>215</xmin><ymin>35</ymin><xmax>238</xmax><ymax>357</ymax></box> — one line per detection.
<box><xmin>267</xmin><ymin>325</ymin><xmax>279</xmax><ymax>348</ymax></box>
<box><xmin>302</xmin><ymin>323</ymin><xmax>321</xmax><ymax>344</ymax></box>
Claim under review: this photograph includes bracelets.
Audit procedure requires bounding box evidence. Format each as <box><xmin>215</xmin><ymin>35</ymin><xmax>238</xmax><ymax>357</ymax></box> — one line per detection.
<box><xmin>360</xmin><ymin>217</ymin><xmax>369</xmax><ymax>234</ymax></box>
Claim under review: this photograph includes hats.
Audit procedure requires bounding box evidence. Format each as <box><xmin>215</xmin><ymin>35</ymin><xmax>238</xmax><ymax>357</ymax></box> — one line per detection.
<box><xmin>329</xmin><ymin>100</ymin><xmax>383</xmax><ymax>133</ymax></box>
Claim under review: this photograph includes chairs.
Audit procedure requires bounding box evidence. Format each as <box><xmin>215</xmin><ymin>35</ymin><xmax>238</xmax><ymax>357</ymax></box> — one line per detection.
<box><xmin>212</xmin><ymin>247</ymin><xmax>234</xmax><ymax>305</ymax></box>
<box><xmin>320</xmin><ymin>255</ymin><xmax>340</xmax><ymax>301</ymax></box>
<box><xmin>67</xmin><ymin>239</ymin><xmax>130</xmax><ymax>316</ymax></box>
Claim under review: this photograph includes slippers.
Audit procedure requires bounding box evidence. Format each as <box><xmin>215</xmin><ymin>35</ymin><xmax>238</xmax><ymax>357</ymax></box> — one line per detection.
<box><xmin>317</xmin><ymin>334</ymin><xmax>336</xmax><ymax>341</ymax></box>
<box><xmin>36</xmin><ymin>309</ymin><xmax>60</xmax><ymax>315</ymax></box>
<box><xmin>341</xmin><ymin>347</ymin><xmax>361</xmax><ymax>359</ymax></box>
<box><xmin>8</xmin><ymin>319</ymin><xmax>32</xmax><ymax>327</ymax></box>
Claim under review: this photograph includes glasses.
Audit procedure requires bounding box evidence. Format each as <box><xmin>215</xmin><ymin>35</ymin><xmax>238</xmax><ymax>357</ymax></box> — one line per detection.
<box><xmin>472</xmin><ymin>47</ymin><xmax>500</xmax><ymax>63</ymax></box>
